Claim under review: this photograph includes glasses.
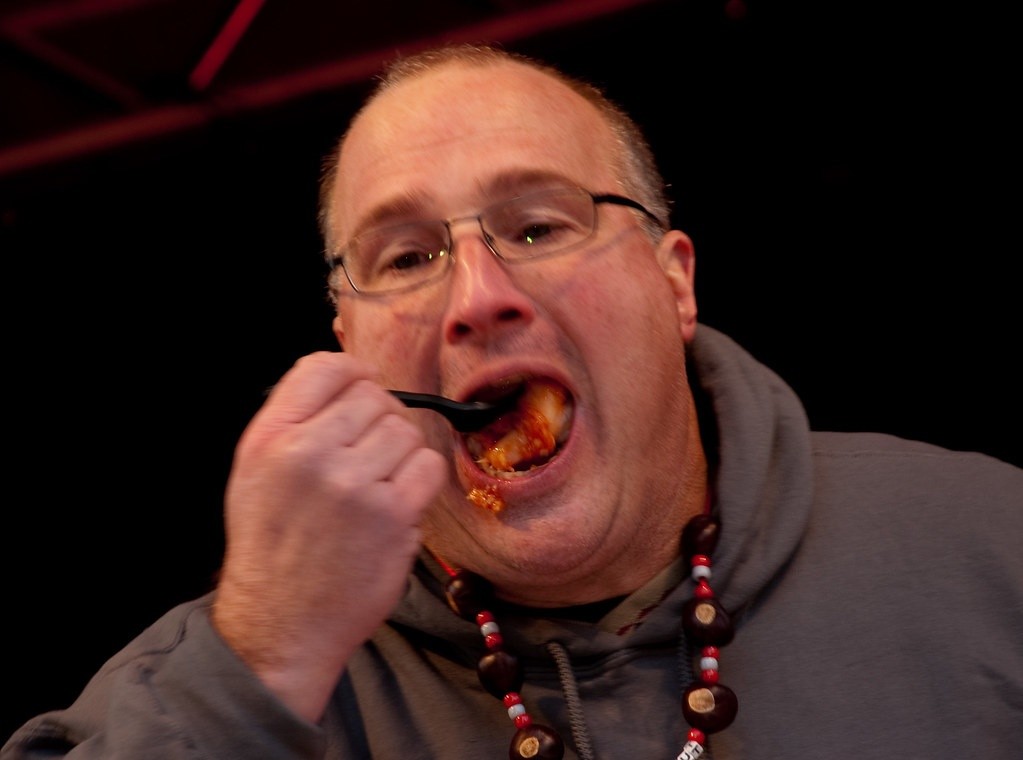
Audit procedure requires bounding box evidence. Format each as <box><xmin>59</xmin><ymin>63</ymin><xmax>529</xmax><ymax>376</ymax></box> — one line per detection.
<box><xmin>329</xmin><ymin>189</ymin><xmax>666</xmax><ymax>296</ymax></box>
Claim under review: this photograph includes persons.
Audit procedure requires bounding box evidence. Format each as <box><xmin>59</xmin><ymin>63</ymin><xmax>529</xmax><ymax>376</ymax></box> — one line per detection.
<box><xmin>0</xmin><ymin>46</ymin><xmax>1023</xmax><ymax>760</ymax></box>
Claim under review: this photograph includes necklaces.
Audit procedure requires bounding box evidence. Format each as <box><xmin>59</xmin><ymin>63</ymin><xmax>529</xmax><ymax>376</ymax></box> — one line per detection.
<box><xmin>438</xmin><ymin>513</ymin><xmax>725</xmax><ymax>760</ymax></box>
